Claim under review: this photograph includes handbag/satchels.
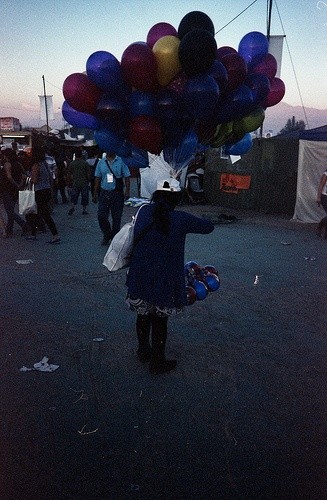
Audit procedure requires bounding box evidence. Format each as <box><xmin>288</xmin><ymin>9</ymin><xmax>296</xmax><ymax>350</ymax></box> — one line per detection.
<box><xmin>18</xmin><ymin>176</ymin><xmax>38</xmax><ymax>216</ymax></box>
<box><xmin>105</xmin><ymin>159</ymin><xmax>125</xmax><ymax>195</ymax></box>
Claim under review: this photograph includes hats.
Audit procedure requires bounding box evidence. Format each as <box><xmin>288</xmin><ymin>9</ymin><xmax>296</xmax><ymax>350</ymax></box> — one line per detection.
<box><xmin>156</xmin><ymin>178</ymin><xmax>182</xmax><ymax>192</ymax></box>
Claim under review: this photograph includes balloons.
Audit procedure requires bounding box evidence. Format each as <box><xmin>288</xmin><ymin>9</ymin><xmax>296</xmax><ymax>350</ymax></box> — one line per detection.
<box><xmin>62</xmin><ymin>12</ymin><xmax>285</xmax><ymax>168</ymax></box>
<box><xmin>184</xmin><ymin>262</ymin><xmax>221</xmax><ymax>306</ymax></box>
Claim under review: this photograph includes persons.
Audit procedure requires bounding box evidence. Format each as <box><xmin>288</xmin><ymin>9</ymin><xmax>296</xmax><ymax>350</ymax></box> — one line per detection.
<box><xmin>125</xmin><ymin>176</ymin><xmax>214</xmax><ymax>375</ymax></box>
<box><xmin>316</xmin><ymin>169</ymin><xmax>327</xmax><ymax>234</ymax></box>
<box><xmin>0</xmin><ymin>142</ymin><xmax>131</xmax><ymax>246</ymax></box>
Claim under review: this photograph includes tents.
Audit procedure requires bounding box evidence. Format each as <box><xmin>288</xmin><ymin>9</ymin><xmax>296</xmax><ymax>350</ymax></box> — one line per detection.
<box><xmin>204</xmin><ymin>124</ymin><xmax>327</xmax><ymax>222</ymax></box>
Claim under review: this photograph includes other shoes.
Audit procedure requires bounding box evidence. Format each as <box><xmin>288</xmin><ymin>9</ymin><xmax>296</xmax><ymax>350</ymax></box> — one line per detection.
<box><xmin>137</xmin><ymin>350</ymin><xmax>178</xmax><ymax>368</ymax></box>
<box><xmin>68</xmin><ymin>208</ymin><xmax>75</xmax><ymax>216</ymax></box>
<box><xmin>48</xmin><ymin>238</ymin><xmax>61</xmax><ymax>245</ymax></box>
<box><xmin>25</xmin><ymin>236</ymin><xmax>37</xmax><ymax>242</ymax></box>
<box><xmin>100</xmin><ymin>237</ymin><xmax>110</xmax><ymax>245</ymax></box>
<box><xmin>82</xmin><ymin>211</ymin><xmax>88</xmax><ymax>216</ymax></box>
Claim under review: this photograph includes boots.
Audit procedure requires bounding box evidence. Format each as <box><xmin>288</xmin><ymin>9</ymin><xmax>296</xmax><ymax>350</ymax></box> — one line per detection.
<box><xmin>314</xmin><ymin>218</ymin><xmax>326</xmax><ymax>238</ymax></box>
